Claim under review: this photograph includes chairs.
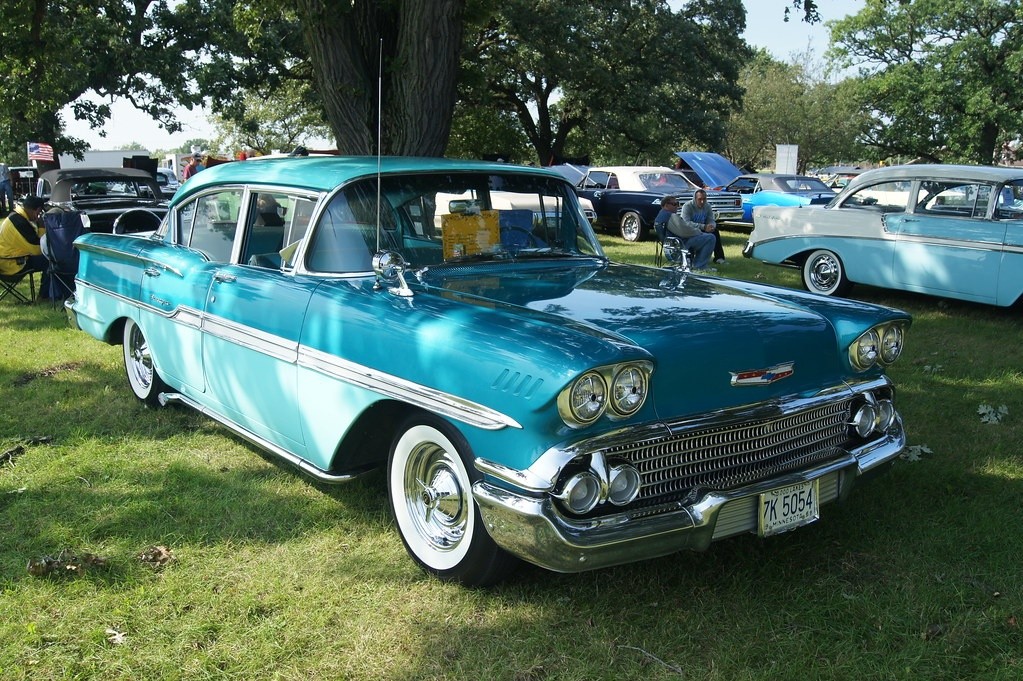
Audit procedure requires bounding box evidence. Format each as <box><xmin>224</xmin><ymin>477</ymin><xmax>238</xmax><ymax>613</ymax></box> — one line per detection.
<box><xmin>0</xmin><ymin>257</ymin><xmax>38</xmax><ymax>307</ymax></box>
<box><xmin>495</xmin><ymin>208</ymin><xmax>553</xmax><ymax>250</ymax></box>
<box><xmin>653</xmin><ymin>222</ymin><xmax>693</xmax><ymax>270</ymax></box>
<box><xmin>194</xmin><ymin>225</ymin><xmax>283</xmax><ymax>270</ymax></box>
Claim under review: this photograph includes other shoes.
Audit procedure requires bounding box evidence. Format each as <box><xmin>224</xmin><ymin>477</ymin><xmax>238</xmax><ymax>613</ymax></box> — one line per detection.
<box><xmin>715</xmin><ymin>259</ymin><xmax>729</xmax><ymax>265</ymax></box>
<box><xmin>704</xmin><ymin>266</ymin><xmax>719</xmax><ymax>272</ymax></box>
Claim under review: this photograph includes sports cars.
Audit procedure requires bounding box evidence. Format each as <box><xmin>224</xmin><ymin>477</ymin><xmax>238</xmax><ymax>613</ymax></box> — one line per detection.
<box><xmin>674</xmin><ymin>152</ymin><xmax>838</xmax><ymax>229</ymax></box>
<box><xmin>75</xmin><ymin>152</ymin><xmax>909</xmax><ymax>587</ymax></box>
<box><xmin>574</xmin><ymin>166</ymin><xmax>745</xmax><ymax>242</ymax></box>
<box><xmin>741</xmin><ymin>163</ymin><xmax>1023</xmax><ymax>319</ymax></box>
<box><xmin>825</xmin><ymin>173</ymin><xmax>966</xmax><ymax>211</ymax></box>
<box><xmin>157</xmin><ymin>168</ymin><xmax>179</xmax><ymax>195</ymax></box>
<box><xmin>36</xmin><ymin>166</ymin><xmax>171</xmax><ymax>237</ymax></box>
<box><xmin>433</xmin><ymin>190</ymin><xmax>599</xmax><ymax>232</ymax></box>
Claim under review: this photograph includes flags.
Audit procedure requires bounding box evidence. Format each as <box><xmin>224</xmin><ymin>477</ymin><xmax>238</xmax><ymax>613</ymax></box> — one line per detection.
<box><xmin>27</xmin><ymin>141</ymin><xmax>55</xmax><ymax>162</ymax></box>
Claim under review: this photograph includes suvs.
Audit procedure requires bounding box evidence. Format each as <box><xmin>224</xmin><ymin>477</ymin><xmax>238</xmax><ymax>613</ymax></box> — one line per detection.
<box><xmin>8</xmin><ymin>166</ymin><xmax>40</xmax><ymax>200</ymax></box>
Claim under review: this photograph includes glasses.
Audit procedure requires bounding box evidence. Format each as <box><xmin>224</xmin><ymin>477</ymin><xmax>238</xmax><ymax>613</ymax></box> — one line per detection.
<box><xmin>668</xmin><ymin>202</ymin><xmax>680</xmax><ymax>206</ymax></box>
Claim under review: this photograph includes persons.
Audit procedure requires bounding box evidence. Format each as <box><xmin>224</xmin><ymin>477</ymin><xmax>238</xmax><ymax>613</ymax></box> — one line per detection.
<box><xmin>0</xmin><ymin>162</ymin><xmax>15</xmax><ymax>214</ymax></box>
<box><xmin>678</xmin><ymin>189</ymin><xmax>733</xmax><ymax>268</ymax></box>
<box><xmin>0</xmin><ymin>194</ymin><xmax>52</xmax><ymax>302</ymax></box>
<box><xmin>653</xmin><ymin>194</ymin><xmax>718</xmax><ymax>273</ymax></box>
<box><xmin>183</xmin><ymin>151</ymin><xmax>202</xmax><ymax>182</ymax></box>
